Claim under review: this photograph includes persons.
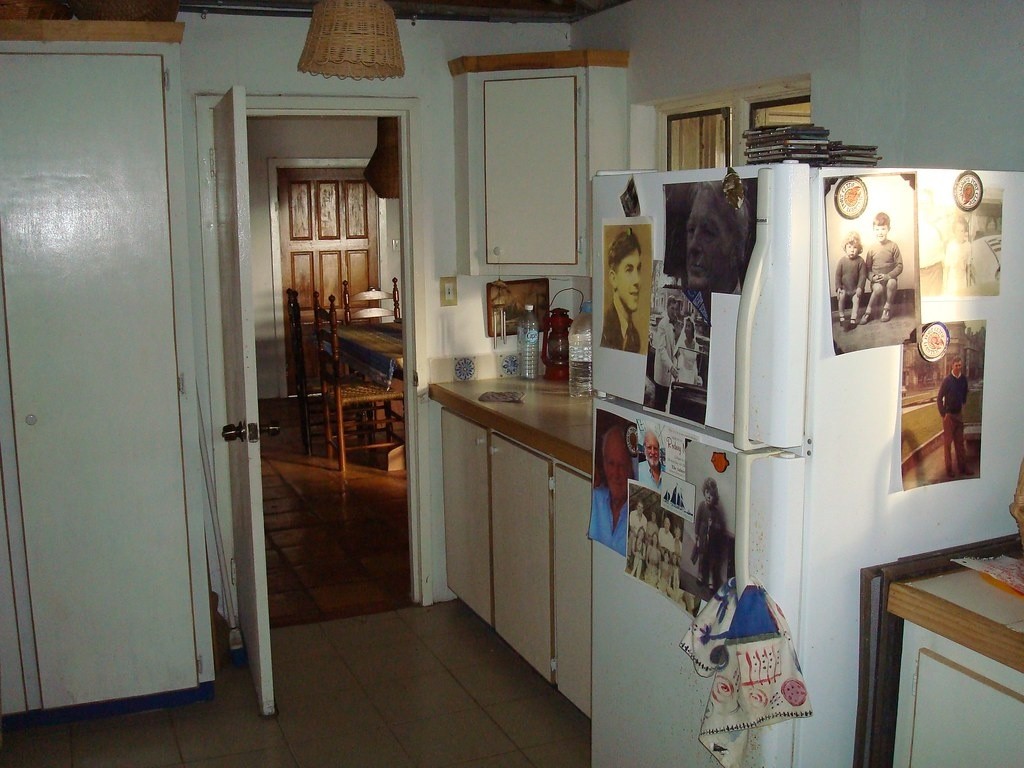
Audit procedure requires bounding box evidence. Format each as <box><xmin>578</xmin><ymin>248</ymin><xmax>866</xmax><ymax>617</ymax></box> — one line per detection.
<box><xmin>588</xmin><ymin>426</ymin><xmax>635</xmax><ymax>557</ymax></box>
<box><xmin>626</xmin><ymin>500</ymin><xmax>695</xmax><ymax>614</ymax></box>
<box><xmin>600</xmin><ymin>231</ymin><xmax>642</xmax><ymax>354</ymax></box>
<box><xmin>834</xmin><ymin>212</ymin><xmax>903</xmax><ymax>329</ymax></box>
<box><xmin>638</xmin><ymin>430</ymin><xmax>665</xmax><ymax>490</ymax></box>
<box><xmin>654</xmin><ymin>295</ymin><xmax>703</xmax><ymax>415</ymax></box>
<box><xmin>695</xmin><ymin>477</ymin><xmax>723</xmax><ymax>592</ymax></box>
<box><xmin>983</xmin><ymin>216</ymin><xmax>1001</xmax><ymax>236</ymax></box>
<box><xmin>686</xmin><ymin>181</ymin><xmax>749</xmax><ymax>294</ymax></box>
<box><xmin>945</xmin><ymin>216</ymin><xmax>972</xmax><ymax>292</ymax></box>
<box><xmin>937</xmin><ymin>355</ymin><xmax>975</xmax><ymax>478</ymax></box>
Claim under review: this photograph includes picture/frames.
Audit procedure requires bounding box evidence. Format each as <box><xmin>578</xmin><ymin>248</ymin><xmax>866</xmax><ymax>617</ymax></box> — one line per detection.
<box><xmin>486</xmin><ymin>278</ymin><xmax>550</xmax><ymax>337</ymax></box>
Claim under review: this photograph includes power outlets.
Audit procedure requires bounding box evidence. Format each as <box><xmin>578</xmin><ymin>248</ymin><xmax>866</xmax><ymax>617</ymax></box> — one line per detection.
<box><xmin>440</xmin><ymin>277</ymin><xmax>457</xmax><ymax>306</ymax></box>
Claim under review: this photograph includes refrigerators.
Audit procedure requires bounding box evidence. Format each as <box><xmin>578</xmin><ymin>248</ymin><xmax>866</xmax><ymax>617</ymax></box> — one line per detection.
<box><xmin>591</xmin><ymin>168</ymin><xmax>1022</xmax><ymax>768</ymax></box>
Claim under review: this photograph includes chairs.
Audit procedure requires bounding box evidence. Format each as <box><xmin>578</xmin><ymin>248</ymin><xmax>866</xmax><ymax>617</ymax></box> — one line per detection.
<box><xmin>286</xmin><ymin>277</ymin><xmax>404</xmax><ymax>472</ymax></box>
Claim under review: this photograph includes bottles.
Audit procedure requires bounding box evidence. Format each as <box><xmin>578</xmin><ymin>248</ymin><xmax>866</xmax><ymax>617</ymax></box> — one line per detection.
<box><xmin>517</xmin><ymin>304</ymin><xmax>539</xmax><ymax>380</ymax></box>
<box><xmin>568</xmin><ymin>300</ymin><xmax>594</xmax><ymax>399</ymax></box>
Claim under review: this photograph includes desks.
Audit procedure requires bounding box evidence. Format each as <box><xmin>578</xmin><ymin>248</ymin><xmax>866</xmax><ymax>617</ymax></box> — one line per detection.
<box><xmin>316</xmin><ymin>323</ymin><xmax>405</xmax><ymax>467</ymax></box>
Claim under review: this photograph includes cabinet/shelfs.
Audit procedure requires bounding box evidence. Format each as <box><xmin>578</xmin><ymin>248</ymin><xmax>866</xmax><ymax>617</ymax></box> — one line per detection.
<box><xmin>449</xmin><ymin>50</ymin><xmax>630</xmax><ymax>277</ymax></box>
<box><xmin>0</xmin><ymin>16</ymin><xmax>216</xmax><ymax>733</ymax></box>
<box><xmin>429</xmin><ymin>375</ymin><xmax>593</xmax><ymax>717</ymax></box>
<box><xmin>887</xmin><ymin>553</ymin><xmax>1024</xmax><ymax>768</ymax></box>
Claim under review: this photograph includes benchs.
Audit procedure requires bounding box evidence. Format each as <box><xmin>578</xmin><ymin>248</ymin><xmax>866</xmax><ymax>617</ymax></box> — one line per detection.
<box><xmin>831</xmin><ymin>289</ymin><xmax>915</xmax><ymax>311</ymax></box>
<box><xmin>964</xmin><ymin>426</ymin><xmax>981</xmax><ymax>474</ymax></box>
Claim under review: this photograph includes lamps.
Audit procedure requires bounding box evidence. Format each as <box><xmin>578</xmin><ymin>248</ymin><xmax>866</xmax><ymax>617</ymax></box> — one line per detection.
<box><xmin>541</xmin><ymin>288</ymin><xmax>584</xmax><ymax>380</ymax></box>
